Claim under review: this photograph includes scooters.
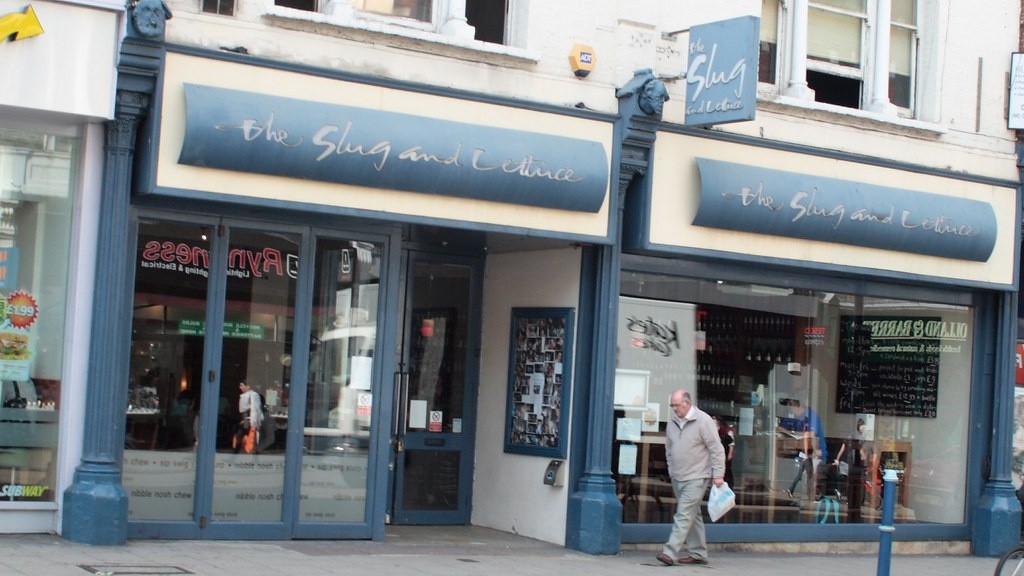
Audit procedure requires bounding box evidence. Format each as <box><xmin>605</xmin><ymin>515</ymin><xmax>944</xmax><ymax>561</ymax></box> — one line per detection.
<box><xmin>839</xmin><ymin>460</ymin><xmax>885</xmax><ymax>510</ymax></box>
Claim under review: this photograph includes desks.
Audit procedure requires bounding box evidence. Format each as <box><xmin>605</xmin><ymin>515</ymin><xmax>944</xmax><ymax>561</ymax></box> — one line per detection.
<box><xmin>614</xmin><ymin>427</ymin><xmax>913</xmax><ymax>524</ymax></box>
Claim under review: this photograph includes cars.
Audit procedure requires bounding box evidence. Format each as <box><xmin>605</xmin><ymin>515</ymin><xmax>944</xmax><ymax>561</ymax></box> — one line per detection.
<box><xmin>898</xmin><ymin>445</ymin><xmax>967</xmax><ymax>523</ymax></box>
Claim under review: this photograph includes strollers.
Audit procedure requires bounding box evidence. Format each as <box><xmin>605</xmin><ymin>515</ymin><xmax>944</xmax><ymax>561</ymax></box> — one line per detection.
<box><xmin>814</xmin><ymin>459</ymin><xmax>842</xmax><ymax>502</ymax></box>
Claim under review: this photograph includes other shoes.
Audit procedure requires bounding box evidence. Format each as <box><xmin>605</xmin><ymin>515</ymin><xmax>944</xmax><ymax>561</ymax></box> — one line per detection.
<box><xmin>782</xmin><ymin>489</ymin><xmax>793</xmax><ymax>499</ymax></box>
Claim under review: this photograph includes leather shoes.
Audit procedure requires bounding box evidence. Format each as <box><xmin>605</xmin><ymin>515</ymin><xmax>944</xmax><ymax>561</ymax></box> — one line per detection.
<box><xmin>678</xmin><ymin>556</ymin><xmax>708</xmax><ymax>564</ymax></box>
<box><xmin>657</xmin><ymin>554</ymin><xmax>674</xmax><ymax>565</ymax></box>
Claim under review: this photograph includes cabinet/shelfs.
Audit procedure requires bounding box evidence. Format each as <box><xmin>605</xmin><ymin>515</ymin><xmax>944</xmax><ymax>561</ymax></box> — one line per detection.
<box><xmin>693</xmin><ymin>308</ymin><xmax>811</xmax><ymax>423</ymax></box>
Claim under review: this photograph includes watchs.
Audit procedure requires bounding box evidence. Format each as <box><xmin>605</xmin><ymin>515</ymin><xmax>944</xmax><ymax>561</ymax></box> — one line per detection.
<box><xmin>791</xmin><ymin>430</ymin><xmax>796</xmax><ymax>434</ymax></box>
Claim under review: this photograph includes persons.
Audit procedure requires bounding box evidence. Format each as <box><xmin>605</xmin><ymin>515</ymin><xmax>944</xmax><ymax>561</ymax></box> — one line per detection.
<box><xmin>133</xmin><ymin>368</ymin><xmax>346</xmax><ymax>454</ymax></box>
<box><xmin>508</xmin><ymin>313</ymin><xmax>567</xmax><ymax>446</ymax></box>
<box><xmin>656</xmin><ymin>388</ymin><xmax>726</xmax><ymax>565</ymax></box>
<box><xmin>710</xmin><ymin>416</ymin><xmax>895</xmax><ymax>509</ymax></box>
<box><xmin>1010</xmin><ymin>394</ymin><xmax>1024</xmax><ymax>489</ymax></box>
<box><xmin>775</xmin><ymin>393</ymin><xmax>828</xmax><ymax>465</ymax></box>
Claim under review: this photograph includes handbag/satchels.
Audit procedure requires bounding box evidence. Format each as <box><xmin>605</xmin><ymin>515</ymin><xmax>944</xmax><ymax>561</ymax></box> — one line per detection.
<box><xmin>707</xmin><ymin>481</ymin><xmax>736</xmax><ymax>522</ymax></box>
<box><xmin>233</xmin><ymin>428</ymin><xmax>255</xmax><ymax>454</ymax></box>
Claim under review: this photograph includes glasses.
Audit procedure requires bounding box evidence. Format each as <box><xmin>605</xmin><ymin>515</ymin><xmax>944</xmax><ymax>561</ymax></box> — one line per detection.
<box><xmin>669</xmin><ymin>403</ymin><xmax>681</xmax><ymax>407</ymax></box>
